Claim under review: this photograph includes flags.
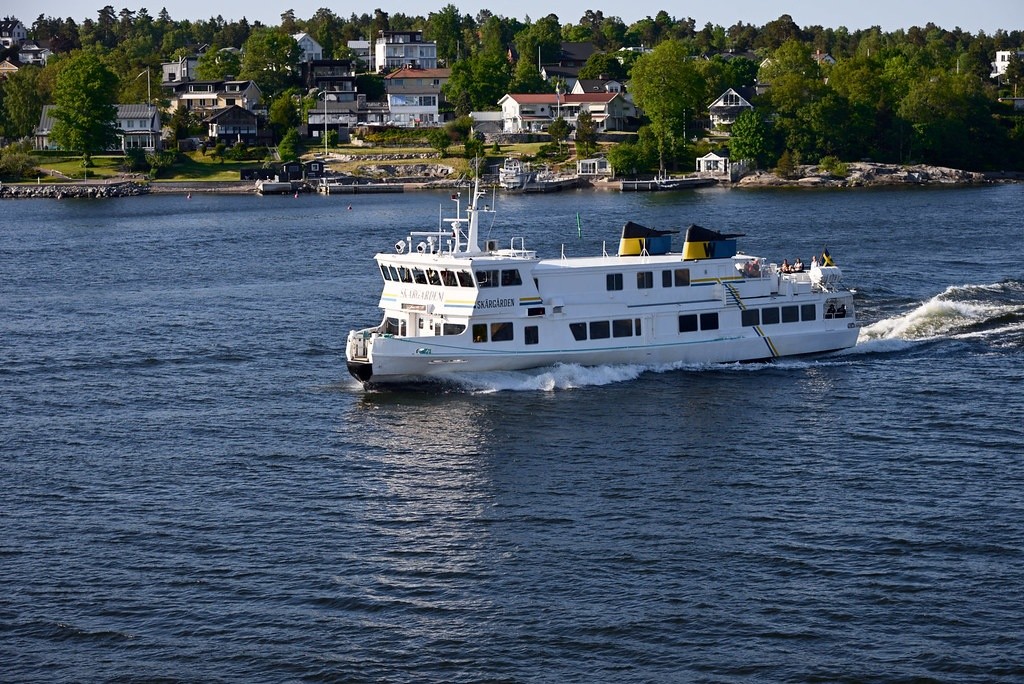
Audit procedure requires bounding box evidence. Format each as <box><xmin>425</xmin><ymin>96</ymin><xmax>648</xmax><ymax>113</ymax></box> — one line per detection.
<box><xmin>819</xmin><ymin>247</ymin><xmax>836</xmax><ymax>266</ymax></box>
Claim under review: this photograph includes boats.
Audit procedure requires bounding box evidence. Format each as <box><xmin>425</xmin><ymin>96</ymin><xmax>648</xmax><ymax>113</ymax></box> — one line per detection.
<box><xmin>498</xmin><ymin>157</ymin><xmax>533</xmax><ymax>190</ymax></box>
<box><xmin>343</xmin><ymin>150</ymin><xmax>862</xmax><ymax>386</ymax></box>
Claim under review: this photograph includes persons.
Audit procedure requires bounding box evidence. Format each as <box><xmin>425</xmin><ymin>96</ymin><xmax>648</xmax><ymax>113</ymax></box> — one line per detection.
<box><xmin>811</xmin><ymin>256</ymin><xmax>820</xmax><ymax>270</ymax></box>
<box><xmin>782</xmin><ymin>258</ymin><xmax>804</xmax><ymax>273</ymax></box>
